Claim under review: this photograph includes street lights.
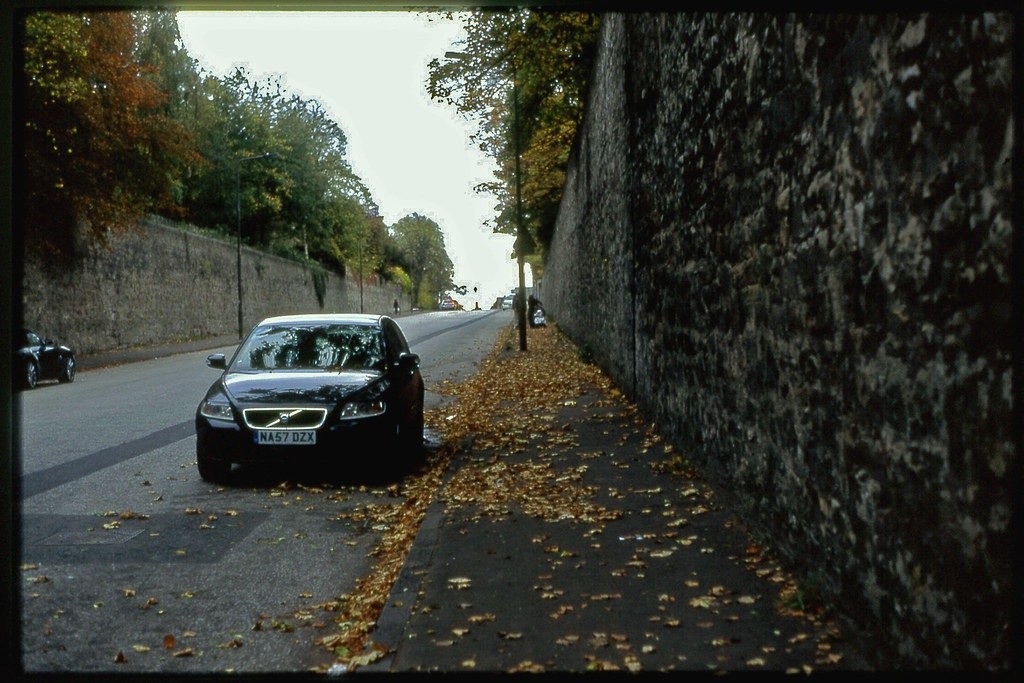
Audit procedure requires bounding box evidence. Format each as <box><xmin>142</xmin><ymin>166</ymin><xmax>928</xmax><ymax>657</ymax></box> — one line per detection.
<box><xmin>358</xmin><ymin>207</ymin><xmax>379</xmax><ymax>313</ymax></box>
<box><xmin>237</xmin><ymin>152</ymin><xmax>274</xmax><ymax>342</ymax></box>
<box><xmin>443</xmin><ymin>48</ymin><xmax>529</xmax><ymax>351</ymax></box>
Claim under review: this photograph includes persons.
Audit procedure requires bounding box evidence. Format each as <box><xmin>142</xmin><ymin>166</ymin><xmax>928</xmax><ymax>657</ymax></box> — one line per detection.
<box><xmin>526</xmin><ymin>294</ymin><xmax>537</xmax><ymax>327</ymax></box>
<box><xmin>393</xmin><ymin>298</ymin><xmax>399</xmax><ymax>314</ymax></box>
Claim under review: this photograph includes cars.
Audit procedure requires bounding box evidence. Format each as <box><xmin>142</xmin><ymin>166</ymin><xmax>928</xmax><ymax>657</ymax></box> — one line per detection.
<box><xmin>441</xmin><ymin>300</ymin><xmax>455</xmax><ymax>310</ymax></box>
<box><xmin>501</xmin><ymin>297</ymin><xmax>513</xmax><ymax>310</ymax></box>
<box><xmin>194</xmin><ymin>314</ymin><xmax>425</xmax><ymax>485</ymax></box>
<box><xmin>12</xmin><ymin>328</ymin><xmax>76</xmax><ymax>388</ymax></box>
